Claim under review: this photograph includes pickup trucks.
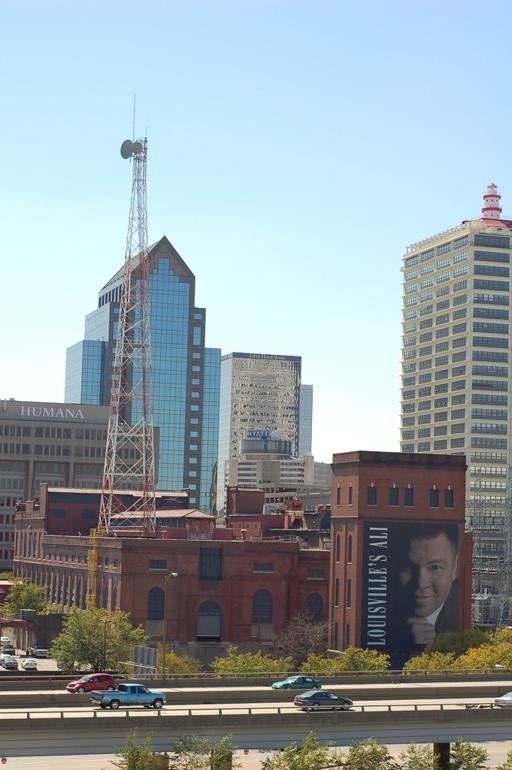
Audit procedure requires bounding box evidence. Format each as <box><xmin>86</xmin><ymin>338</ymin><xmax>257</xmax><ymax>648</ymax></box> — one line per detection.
<box><xmin>89</xmin><ymin>683</ymin><xmax>168</xmax><ymax>711</ymax></box>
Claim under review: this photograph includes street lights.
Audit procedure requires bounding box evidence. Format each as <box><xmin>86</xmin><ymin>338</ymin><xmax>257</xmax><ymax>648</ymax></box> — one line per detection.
<box><xmin>164</xmin><ymin>572</ymin><xmax>179</xmax><ymax>641</ymax></box>
<box><xmin>103</xmin><ymin>620</ymin><xmax>112</xmax><ymax>665</ymax></box>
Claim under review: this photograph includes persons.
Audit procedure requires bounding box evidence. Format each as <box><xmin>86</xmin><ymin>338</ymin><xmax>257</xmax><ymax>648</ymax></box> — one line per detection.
<box><xmin>390</xmin><ymin>523</ymin><xmax>460</xmax><ymax>665</ymax></box>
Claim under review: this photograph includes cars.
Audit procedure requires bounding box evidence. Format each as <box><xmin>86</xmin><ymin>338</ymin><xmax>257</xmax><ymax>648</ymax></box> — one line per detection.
<box><xmin>271</xmin><ymin>675</ymin><xmax>321</xmax><ymax>692</ymax></box>
<box><xmin>293</xmin><ymin>690</ymin><xmax>354</xmax><ymax>712</ymax></box>
<box><xmin>0</xmin><ymin>637</ymin><xmax>49</xmax><ymax>671</ymax></box>
<box><xmin>66</xmin><ymin>673</ymin><xmax>118</xmax><ymax>693</ymax></box>
<box><xmin>494</xmin><ymin>692</ymin><xmax>511</xmax><ymax>708</ymax></box>
